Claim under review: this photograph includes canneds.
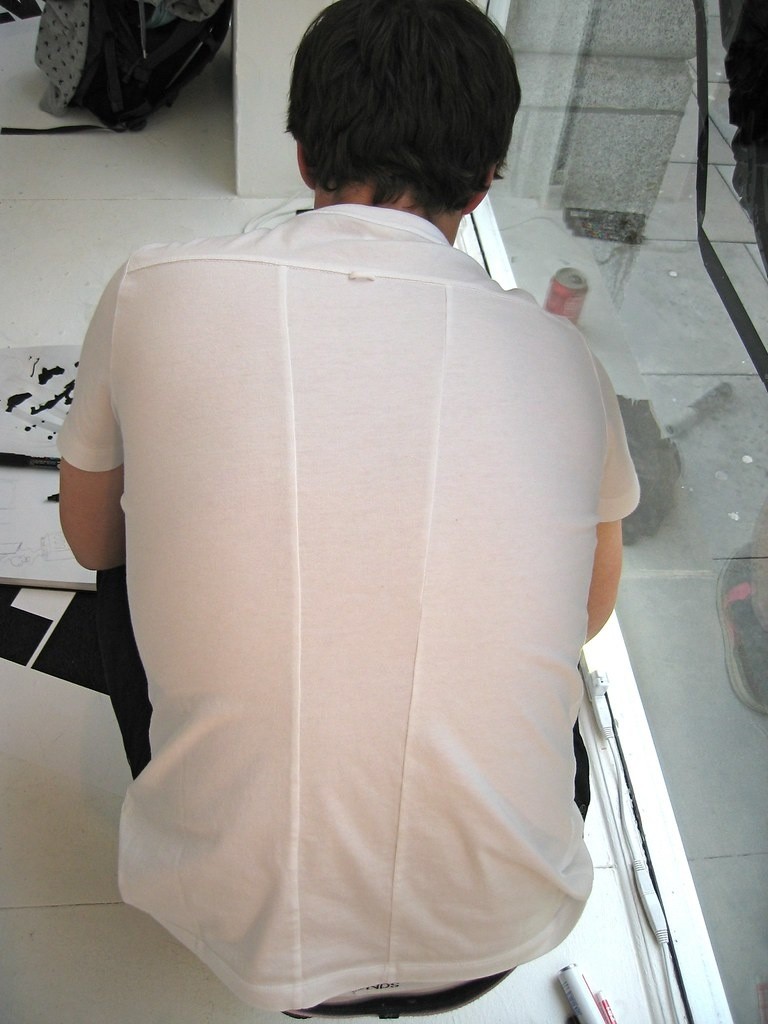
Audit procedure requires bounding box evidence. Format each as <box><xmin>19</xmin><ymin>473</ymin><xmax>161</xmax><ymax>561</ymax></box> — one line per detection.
<box><xmin>544</xmin><ymin>267</ymin><xmax>588</xmax><ymax>324</ymax></box>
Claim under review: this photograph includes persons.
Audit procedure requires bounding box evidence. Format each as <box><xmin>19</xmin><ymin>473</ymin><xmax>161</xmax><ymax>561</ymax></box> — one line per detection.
<box><xmin>58</xmin><ymin>0</ymin><xmax>640</xmax><ymax>1020</ymax></box>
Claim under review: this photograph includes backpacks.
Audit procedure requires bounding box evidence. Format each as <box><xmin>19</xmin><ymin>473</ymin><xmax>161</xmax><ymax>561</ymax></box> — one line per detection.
<box><xmin>68</xmin><ymin>0</ymin><xmax>235</xmax><ymax>134</ymax></box>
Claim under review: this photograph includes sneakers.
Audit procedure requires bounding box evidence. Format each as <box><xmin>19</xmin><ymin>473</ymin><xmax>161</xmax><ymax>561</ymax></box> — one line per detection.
<box><xmin>716</xmin><ymin>543</ymin><xmax>768</xmax><ymax>713</ymax></box>
